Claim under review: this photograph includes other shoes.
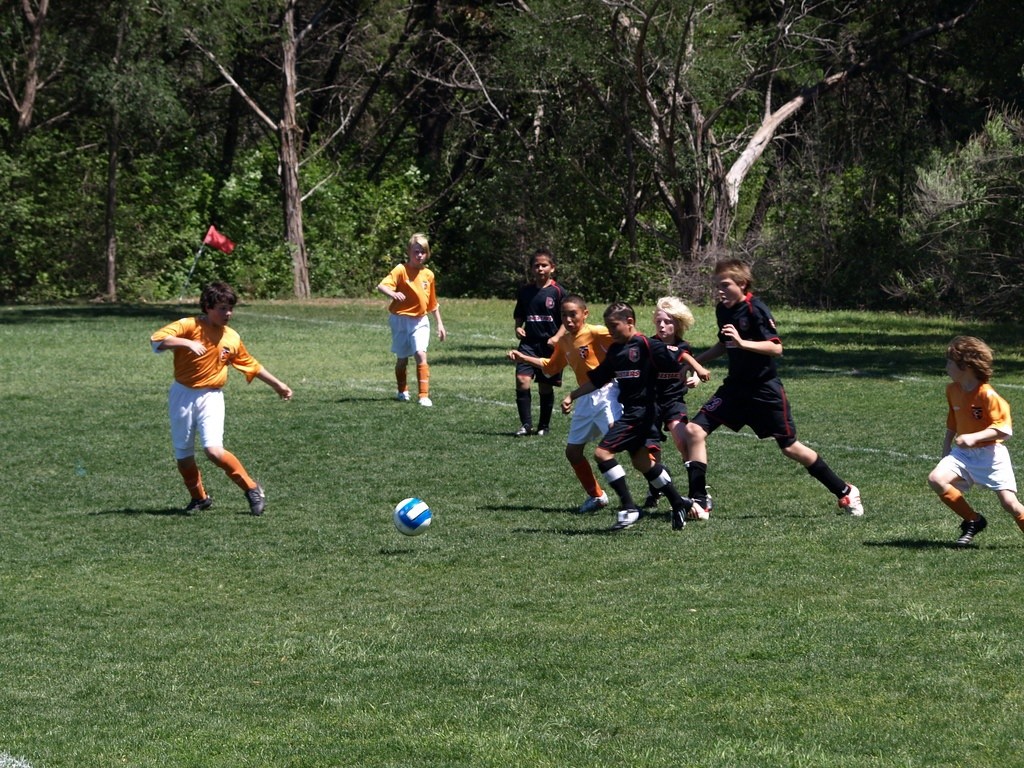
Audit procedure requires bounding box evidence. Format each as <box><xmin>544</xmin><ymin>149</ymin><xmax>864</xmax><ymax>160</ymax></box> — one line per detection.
<box><xmin>706</xmin><ymin>492</ymin><xmax>713</xmax><ymax>509</ymax></box>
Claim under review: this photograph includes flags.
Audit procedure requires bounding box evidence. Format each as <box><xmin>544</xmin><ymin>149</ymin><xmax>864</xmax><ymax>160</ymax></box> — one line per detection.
<box><xmin>203</xmin><ymin>225</ymin><xmax>236</xmax><ymax>254</ymax></box>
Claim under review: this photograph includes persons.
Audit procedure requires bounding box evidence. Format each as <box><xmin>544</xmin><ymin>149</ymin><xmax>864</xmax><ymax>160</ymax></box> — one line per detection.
<box><xmin>506</xmin><ymin>294</ymin><xmax>693</xmax><ymax>530</ymax></box>
<box><xmin>642</xmin><ymin>296</ymin><xmax>712</xmax><ymax>512</ymax></box>
<box><xmin>514</xmin><ymin>250</ymin><xmax>570</xmax><ymax>436</ymax></box>
<box><xmin>670</xmin><ymin>259</ymin><xmax>864</xmax><ymax>517</ymax></box>
<box><xmin>928</xmin><ymin>336</ymin><xmax>1024</xmax><ymax>544</ymax></box>
<box><xmin>377</xmin><ymin>233</ymin><xmax>445</xmax><ymax>407</ymax></box>
<box><xmin>151</xmin><ymin>282</ymin><xmax>292</xmax><ymax>515</ymax></box>
<box><xmin>562</xmin><ymin>302</ymin><xmax>711</xmax><ymax>530</ymax></box>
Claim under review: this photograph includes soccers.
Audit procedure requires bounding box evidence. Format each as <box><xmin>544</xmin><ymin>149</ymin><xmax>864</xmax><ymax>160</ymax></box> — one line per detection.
<box><xmin>392</xmin><ymin>498</ymin><xmax>432</xmax><ymax>536</ymax></box>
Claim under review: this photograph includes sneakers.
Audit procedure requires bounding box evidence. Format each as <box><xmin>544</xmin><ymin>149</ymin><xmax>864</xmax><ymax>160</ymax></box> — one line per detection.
<box><xmin>417</xmin><ymin>396</ymin><xmax>432</xmax><ymax>407</ymax></box>
<box><xmin>669</xmin><ymin>496</ymin><xmax>711</xmax><ymax>521</ymax></box>
<box><xmin>537</xmin><ymin>427</ymin><xmax>549</xmax><ymax>437</ymax></box>
<box><xmin>609</xmin><ymin>506</ymin><xmax>643</xmax><ymax>531</ymax></box>
<box><xmin>398</xmin><ymin>389</ymin><xmax>410</xmax><ymax>401</ymax></box>
<box><xmin>244</xmin><ymin>483</ymin><xmax>266</xmax><ymax>516</ymax></box>
<box><xmin>641</xmin><ymin>495</ymin><xmax>658</xmax><ymax>515</ymax></box>
<box><xmin>838</xmin><ymin>483</ymin><xmax>864</xmax><ymax>517</ymax></box>
<box><xmin>577</xmin><ymin>490</ymin><xmax>610</xmax><ymax>513</ymax></box>
<box><xmin>516</xmin><ymin>426</ymin><xmax>532</xmax><ymax>437</ymax></box>
<box><xmin>669</xmin><ymin>496</ymin><xmax>693</xmax><ymax>532</ymax></box>
<box><xmin>957</xmin><ymin>513</ymin><xmax>988</xmax><ymax>545</ymax></box>
<box><xmin>183</xmin><ymin>496</ymin><xmax>213</xmax><ymax>513</ymax></box>
